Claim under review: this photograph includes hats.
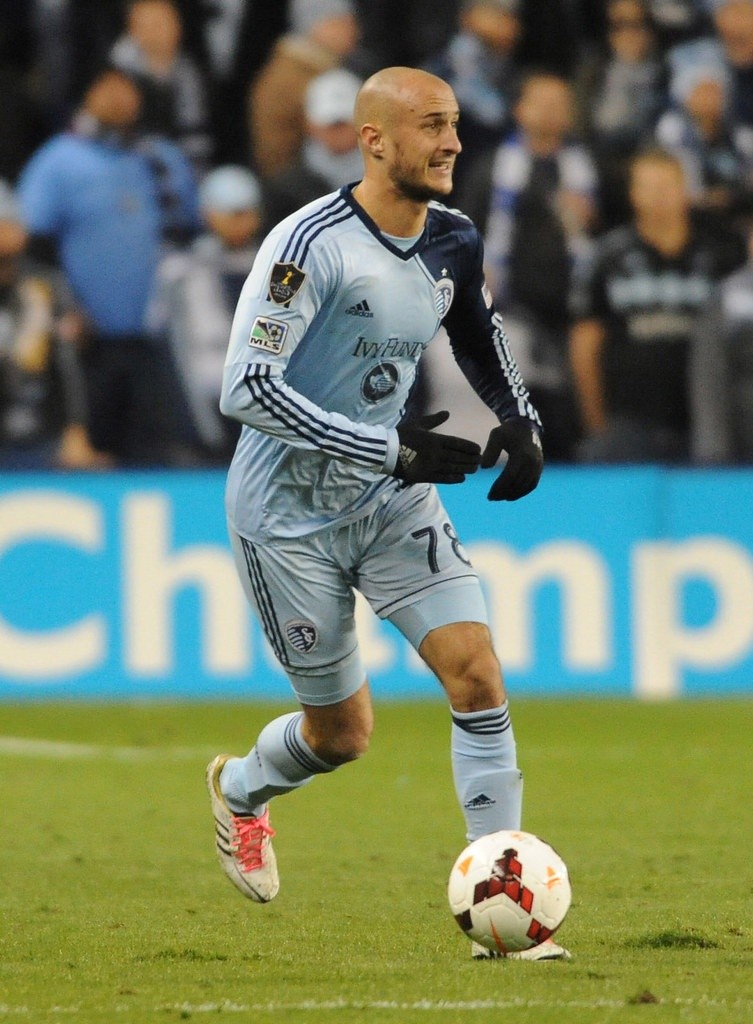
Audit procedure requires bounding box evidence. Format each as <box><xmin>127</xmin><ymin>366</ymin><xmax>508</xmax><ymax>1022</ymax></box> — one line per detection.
<box><xmin>200</xmin><ymin>163</ymin><xmax>262</xmax><ymax>212</ymax></box>
<box><xmin>303</xmin><ymin>70</ymin><xmax>363</xmax><ymax>129</ymax></box>
<box><xmin>668</xmin><ymin>38</ymin><xmax>734</xmax><ymax>103</ymax></box>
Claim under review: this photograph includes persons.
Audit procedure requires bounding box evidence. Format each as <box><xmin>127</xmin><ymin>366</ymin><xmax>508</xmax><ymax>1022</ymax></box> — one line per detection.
<box><xmin>0</xmin><ymin>0</ymin><xmax>753</xmax><ymax>468</ymax></box>
<box><xmin>205</xmin><ymin>66</ymin><xmax>572</xmax><ymax>962</ymax></box>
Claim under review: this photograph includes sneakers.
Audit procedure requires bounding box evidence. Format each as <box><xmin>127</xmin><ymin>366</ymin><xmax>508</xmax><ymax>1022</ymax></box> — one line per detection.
<box><xmin>207</xmin><ymin>754</ymin><xmax>279</xmax><ymax>903</ymax></box>
<box><xmin>472</xmin><ymin>938</ymin><xmax>571</xmax><ymax>961</ymax></box>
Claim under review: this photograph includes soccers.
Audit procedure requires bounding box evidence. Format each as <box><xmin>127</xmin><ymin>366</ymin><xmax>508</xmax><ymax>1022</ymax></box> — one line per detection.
<box><xmin>447</xmin><ymin>829</ymin><xmax>573</xmax><ymax>954</ymax></box>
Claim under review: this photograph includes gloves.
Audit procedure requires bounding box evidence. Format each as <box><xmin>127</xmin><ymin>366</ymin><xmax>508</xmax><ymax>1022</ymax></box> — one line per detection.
<box><xmin>481</xmin><ymin>416</ymin><xmax>543</xmax><ymax>501</ymax></box>
<box><xmin>391</xmin><ymin>410</ymin><xmax>481</xmax><ymax>484</ymax></box>
